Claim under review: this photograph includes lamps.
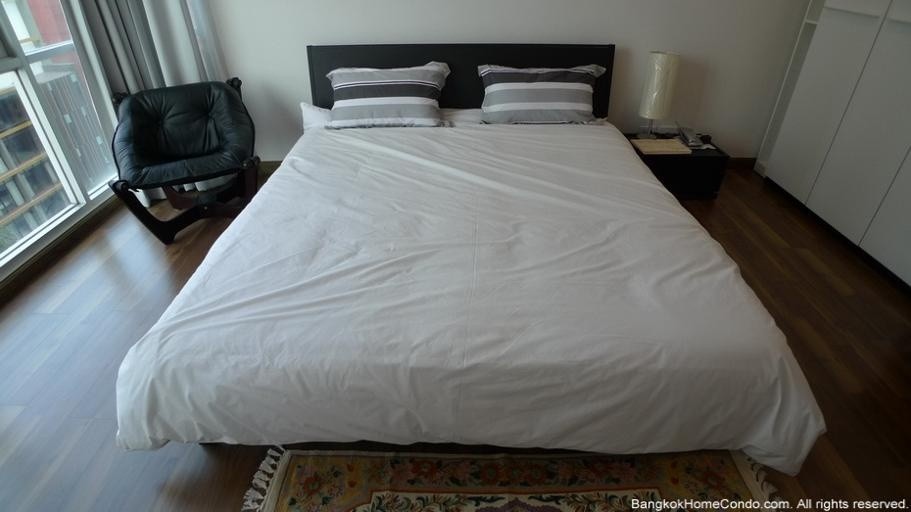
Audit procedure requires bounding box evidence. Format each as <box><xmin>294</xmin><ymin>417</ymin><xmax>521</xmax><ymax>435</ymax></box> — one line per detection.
<box><xmin>638</xmin><ymin>52</ymin><xmax>680</xmax><ymax>143</ymax></box>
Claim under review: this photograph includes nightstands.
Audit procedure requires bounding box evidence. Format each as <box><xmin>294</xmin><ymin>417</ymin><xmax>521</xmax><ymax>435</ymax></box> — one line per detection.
<box><xmin>622</xmin><ymin>132</ymin><xmax>731</xmax><ymax>204</ymax></box>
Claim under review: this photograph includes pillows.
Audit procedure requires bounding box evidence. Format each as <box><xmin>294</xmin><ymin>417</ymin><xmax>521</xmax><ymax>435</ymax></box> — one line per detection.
<box><xmin>476</xmin><ymin>64</ymin><xmax>607</xmax><ymax>125</ymax></box>
<box><xmin>325</xmin><ymin>60</ymin><xmax>451</xmax><ymax>128</ymax></box>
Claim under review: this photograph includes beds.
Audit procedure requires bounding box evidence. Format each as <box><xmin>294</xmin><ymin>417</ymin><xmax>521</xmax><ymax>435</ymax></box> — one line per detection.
<box><xmin>117</xmin><ymin>44</ymin><xmax>829</xmax><ymax>478</ymax></box>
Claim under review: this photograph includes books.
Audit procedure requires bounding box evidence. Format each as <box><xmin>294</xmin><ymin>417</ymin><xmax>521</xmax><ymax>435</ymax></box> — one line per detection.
<box><xmin>630</xmin><ymin>138</ymin><xmax>693</xmax><ymax>155</ymax></box>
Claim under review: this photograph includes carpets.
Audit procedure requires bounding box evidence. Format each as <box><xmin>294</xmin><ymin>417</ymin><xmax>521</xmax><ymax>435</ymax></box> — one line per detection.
<box><xmin>238</xmin><ymin>439</ymin><xmax>790</xmax><ymax>511</ymax></box>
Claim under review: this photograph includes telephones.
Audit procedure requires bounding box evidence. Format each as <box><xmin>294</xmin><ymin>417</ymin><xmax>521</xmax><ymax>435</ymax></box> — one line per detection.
<box><xmin>678</xmin><ymin>127</ymin><xmax>703</xmax><ymax>146</ymax></box>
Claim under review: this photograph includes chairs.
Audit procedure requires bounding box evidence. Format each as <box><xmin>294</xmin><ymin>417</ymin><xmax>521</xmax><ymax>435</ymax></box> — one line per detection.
<box><xmin>107</xmin><ymin>76</ymin><xmax>261</xmax><ymax>244</ymax></box>
